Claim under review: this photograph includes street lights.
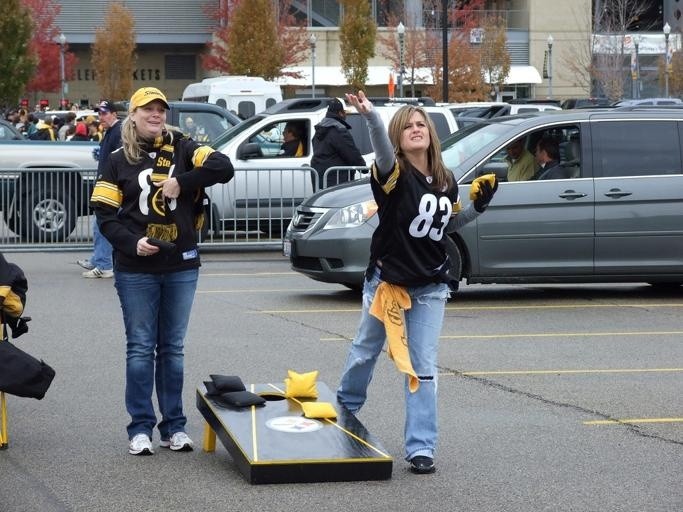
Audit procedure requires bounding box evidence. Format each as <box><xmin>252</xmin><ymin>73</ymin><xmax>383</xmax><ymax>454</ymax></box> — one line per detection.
<box><xmin>58</xmin><ymin>32</ymin><xmax>67</xmax><ymax>109</ymax></box>
<box><xmin>663</xmin><ymin>22</ymin><xmax>671</xmax><ymax>97</ymax></box>
<box><xmin>60</xmin><ymin>33</ymin><xmax>65</xmax><ymax>109</ymax></box>
<box><xmin>309</xmin><ymin>33</ymin><xmax>318</xmax><ymax>98</ymax></box>
<box><xmin>310</xmin><ymin>34</ymin><xmax>316</xmax><ymax>98</ymax></box>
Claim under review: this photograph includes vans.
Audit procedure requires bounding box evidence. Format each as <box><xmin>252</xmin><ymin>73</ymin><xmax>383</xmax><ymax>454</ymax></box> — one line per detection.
<box><xmin>282</xmin><ymin>110</ymin><xmax>683</xmax><ymax>302</ymax></box>
<box><xmin>183</xmin><ymin>75</ymin><xmax>282</xmax><ymax>119</ymax></box>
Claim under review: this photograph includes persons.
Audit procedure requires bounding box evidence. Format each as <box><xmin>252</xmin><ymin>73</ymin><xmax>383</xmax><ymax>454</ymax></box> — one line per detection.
<box><xmin>6</xmin><ymin>103</ymin><xmax>77</xmax><ymax>142</ymax></box>
<box><xmin>506</xmin><ymin>140</ymin><xmax>541</xmax><ymax>182</ymax></box>
<box><xmin>527</xmin><ymin>138</ymin><xmax>567</xmax><ymax>179</ymax></box>
<box><xmin>180</xmin><ymin>117</ymin><xmax>197</xmax><ymax>139</ymax></box>
<box><xmin>345</xmin><ymin>90</ymin><xmax>499</xmax><ymax>474</ymax></box>
<box><xmin>77</xmin><ymin>100</ymin><xmax>125</xmax><ymax>279</ymax></box>
<box><xmin>277</xmin><ymin>121</ymin><xmax>303</xmax><ymax>157</ymax></box>
<box><xmin>311</xmin><ymin>97</ymin><xmax>369</xmax><ymax>195</ymax></box>
<box><xmin>88</xmin><ymin>87</ymin><xmax>235</xmax><ymax>457</ymax></box>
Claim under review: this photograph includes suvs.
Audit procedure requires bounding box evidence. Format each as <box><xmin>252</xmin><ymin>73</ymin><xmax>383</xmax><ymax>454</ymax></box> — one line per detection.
<box><xmin>629</xmin><ymin>97</ymin><xmax>682</xmax><ymax>106</ymax></box>
<box><xmin>437</xmin><ymin>99</ymin><xmax>561</xmax><ymax>129</ymax></box>
<box><xmin>203</xmin><ymin>95</ymin><xmax>460</xmax><ymax>239</ymax></box>
<box><xmin>562</xmin><ymin>97</ymin><xmax>614</xmax><ymax>110</ymax></box>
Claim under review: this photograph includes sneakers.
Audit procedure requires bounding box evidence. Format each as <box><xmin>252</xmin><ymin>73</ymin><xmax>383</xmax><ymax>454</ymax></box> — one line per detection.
<box><xmin>130</xmin><ymin>434</ymin><xmax>155</xmax><ymax>456</ymax></box>
<box><xmin>82</xmin><ymin>266</ymin><xmax>115</xmax><ymax>279</ymax></box>
<box><xmin>77</xmin><ymin>258</ymin><xmax>96</xmax><ymax>270</ymax></box>
<box><xmin>160</xmin><ymin>430</ymin><xmax>195</xmax><ymax>452</ymax></box>
<box><xmin>410</xmin><ymin>455</ymin><xmax>436</xmax><ymax>475</ymax></box>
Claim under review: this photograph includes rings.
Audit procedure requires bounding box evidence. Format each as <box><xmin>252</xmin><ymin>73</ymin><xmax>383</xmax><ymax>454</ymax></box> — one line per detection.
<box><xmin>143</xmin><ymin>253</ymin><xmax>148</xmax><ymax>256</ymax></box>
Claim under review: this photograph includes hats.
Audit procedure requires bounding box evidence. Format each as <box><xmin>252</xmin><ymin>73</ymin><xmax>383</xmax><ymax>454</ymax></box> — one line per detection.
<box><xmin>95</xmin><ymin>101</ymin><xmax>119</xmax><ymax>114</ymax></box>
<box><xmin>329</xmin><ymin>97</ymin><xmax>351</xmax><ymax>112</ymax></box>
<box><xmin>129</xmin><ymin>87</ymin><xmax>171</xmax><ymax>111</ymax></box>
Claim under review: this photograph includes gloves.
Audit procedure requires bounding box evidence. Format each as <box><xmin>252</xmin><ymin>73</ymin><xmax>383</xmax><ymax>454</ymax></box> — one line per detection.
<box><xmin>475</xmin><ymin>180</ymin><xmax>500</xmax><ymax>215</ymax></box>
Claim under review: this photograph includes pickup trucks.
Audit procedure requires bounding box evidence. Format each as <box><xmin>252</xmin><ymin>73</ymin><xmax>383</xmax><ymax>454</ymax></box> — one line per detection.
<box><xmin>0</xmin><ymin>101</ymin><xmax>285</xmax><ymax>242</ymax></box>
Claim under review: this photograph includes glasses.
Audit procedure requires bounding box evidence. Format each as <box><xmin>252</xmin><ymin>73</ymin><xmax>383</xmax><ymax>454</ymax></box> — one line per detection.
<box><xmin>506</xmin><ymin>146</ymin><xmax>519</xmax><ymax>150</ymax></box>
